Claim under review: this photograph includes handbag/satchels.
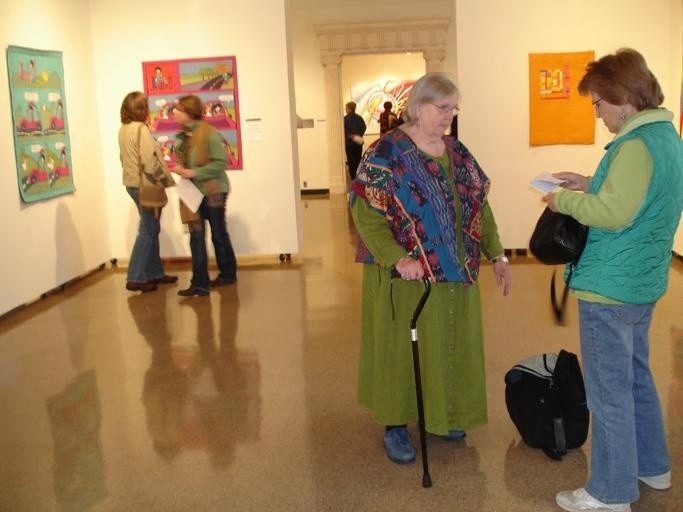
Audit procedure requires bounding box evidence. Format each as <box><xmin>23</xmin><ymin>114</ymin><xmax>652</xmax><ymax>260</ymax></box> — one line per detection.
<box><xmin>139</xmin><ymin>179</ymin><xmax>168</xmax><ymax>208</ymax></box>
<box><xmin>529</xmin><ymin>206</ymin><xmax>589</xmax><ymax>265</ymax></box>
<box><xmin>505</xmin><ymin>349</ymin><xmax>589</xmax><ymax>450</ymax></box>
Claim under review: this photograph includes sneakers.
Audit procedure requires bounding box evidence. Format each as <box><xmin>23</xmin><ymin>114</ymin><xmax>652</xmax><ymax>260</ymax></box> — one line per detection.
<box><xmin>383</xmin><ymin>425</ymin><xmax>416</xmax><ymax>464</ymax></box>
<box><xmin>127</xmin><ymin>280</ymin><xmax>157</xmax><ymax>292</ymax></box>
<box><xmin>555</xmin><ymin>487</ymin><xmax>631</xmax><ymax>512</ymax></box>
<box><xmin>177</xmin><ymin>287</ymin><xmax>209</xmax><ymax>296</ymax></box>
<box><xmin>637</xmin><ymin>470</ymin><xmax>672</xmax><ymax>490</ymax></box>
<box><xmin>209</xmin><ymin>278</ymin><xmax>237</xmax><ymax>287</ymax></box>
<box><xmin>431</xmin><ymin>430</ymin><xmax>466</xmax><ymax>441</ymax></box>
<box><xmin>149</xmin><ymin>275</ymin><xmax>178</xmax><ymax>283</ymax></box>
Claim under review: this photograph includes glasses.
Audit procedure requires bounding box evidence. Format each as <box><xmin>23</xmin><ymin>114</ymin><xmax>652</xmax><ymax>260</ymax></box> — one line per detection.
<box><xmin>429</xmin><ymin>101</ymin><xmax>460</xmax><ymax>118</ymax></box>
<box><xmin>589</xmin><ymin>97</ymin><xmax>604</xmax><ymax>114</ymax></box>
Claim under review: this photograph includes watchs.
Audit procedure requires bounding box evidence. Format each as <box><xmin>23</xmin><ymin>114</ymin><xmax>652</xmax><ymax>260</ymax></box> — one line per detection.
<box><xmin>492</xmin><ymin>256</ymin><xmax>509</xmax><ymax>266</ymax></box>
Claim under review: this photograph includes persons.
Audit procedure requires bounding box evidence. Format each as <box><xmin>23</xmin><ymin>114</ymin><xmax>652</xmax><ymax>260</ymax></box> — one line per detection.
<box><xmin>343</xmin><ymin>100</ymin><xmax>367</xmax><ymax>179</ymax></box>
<box><xmin>347</xmin><ymin>72</ymin><xmax>512</xmax><ymax>466</ymax></box>
<box><xmin>377</xmin><ymin>100</ymin><xmax>397</xmax><ymax>139</ymax></box>
<box><xmin>539</xmin><ymin>45</ymin><xmax>683</xmax><ymax>512</ymax></box>
<box><xmin>392</xmin><ymin>111</ymin><xmax>408</xmax><ymax>129</ymax></box>
<box><xmin>449</xmin><ymin>114</ymin><xmax>457</xmax><ymax>140</ymax></box>
<box><xmin>117</xmin><ymin>91</ymin><xmax>178</xmax><ymax>294</ymax></box>
<box><xmin>166</xmin><ymin>95</ymin><xmax>238</xmax><ymax>296</ymax></box>
